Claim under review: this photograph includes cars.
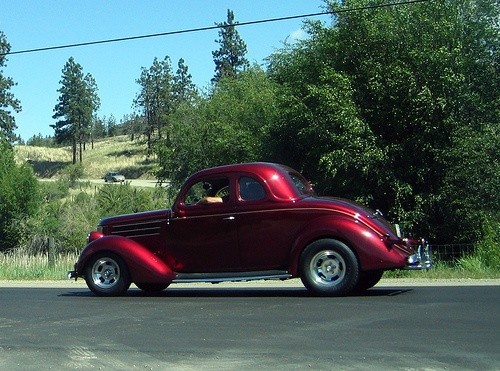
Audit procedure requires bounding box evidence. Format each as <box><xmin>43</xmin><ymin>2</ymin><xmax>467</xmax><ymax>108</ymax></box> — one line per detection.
<box><xmin>66</xmin><ymin>162</ymin><xmax>432</xmax><ymax>299</ymax></box>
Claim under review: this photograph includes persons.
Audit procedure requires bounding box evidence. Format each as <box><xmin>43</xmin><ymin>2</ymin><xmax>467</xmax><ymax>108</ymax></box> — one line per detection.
<box><xmin>191</xmin><ymin>194</ymin><xmax>228</xmax><ymax>206</ymax></box>
